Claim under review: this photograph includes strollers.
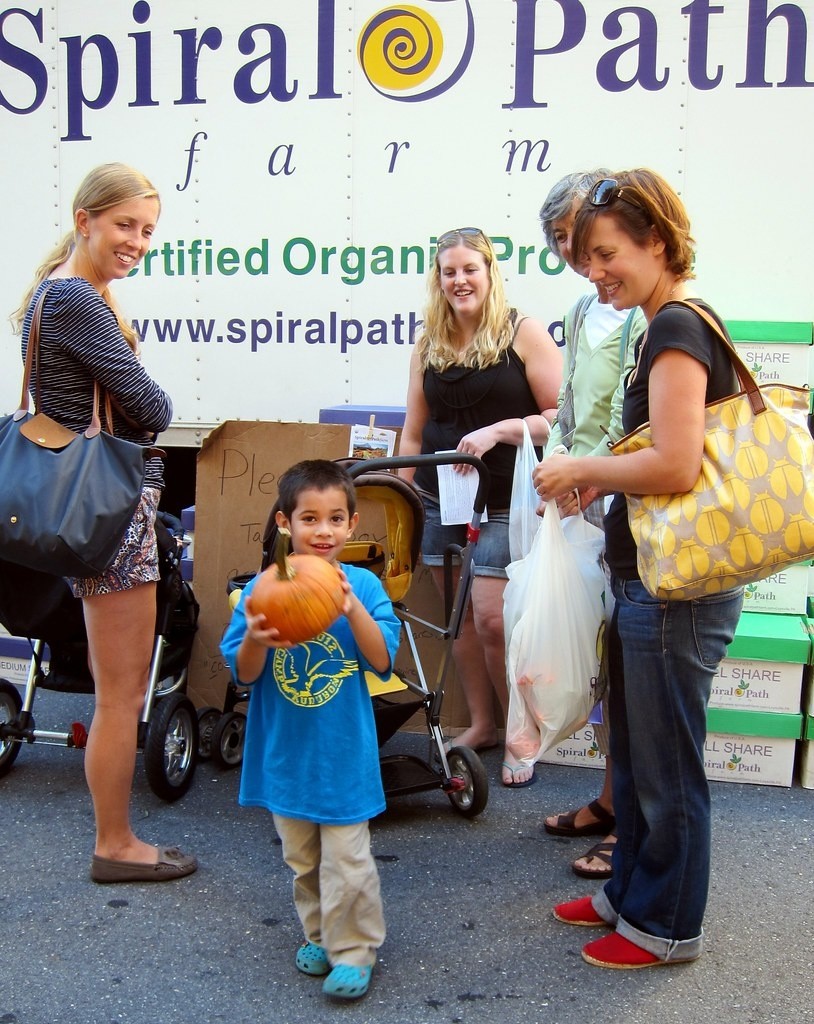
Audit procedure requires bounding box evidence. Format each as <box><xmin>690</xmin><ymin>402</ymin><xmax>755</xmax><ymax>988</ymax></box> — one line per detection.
<box><xmin>196</xmin><ymin>452</ymin><xmax>490</xmax><ymax>819</ymax></box>
<box><xmin>0</xmin><ymin>512</ymin><xmax>199</xmax><ymax>804</ymax></box>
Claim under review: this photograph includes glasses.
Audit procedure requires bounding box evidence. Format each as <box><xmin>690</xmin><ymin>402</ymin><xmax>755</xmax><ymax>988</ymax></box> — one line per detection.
<box><xmin>588</xmin><ymin>178</ymin><xmax>644</xmax><ymax>211</ymax></box>
<box><xmin>437</xmin><ymin>227</ymin><xmax>489</xmax><ymax>250</ymax></box>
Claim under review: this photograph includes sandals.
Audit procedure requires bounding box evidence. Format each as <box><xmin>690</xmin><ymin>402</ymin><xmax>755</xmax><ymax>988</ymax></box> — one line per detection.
<box><xmin>544</xmin><ymin>798</ymin><xmax>616</xmax><ymax>836</ymax></box>
<box><xmin>571</xmin><ymin>827</ymin><xmax>619</xmax><ymax>878</ymax></box>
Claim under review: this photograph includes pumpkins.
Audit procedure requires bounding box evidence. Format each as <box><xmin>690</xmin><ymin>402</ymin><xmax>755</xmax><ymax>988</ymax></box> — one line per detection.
<box><xmin>249</xmin><ymin>529</ymin><xmax>344</xmax><ymax>643</ymax></box>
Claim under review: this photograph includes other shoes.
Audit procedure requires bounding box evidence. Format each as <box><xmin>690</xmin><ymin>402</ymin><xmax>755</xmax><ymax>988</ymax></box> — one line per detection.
<box><xmin>583</xmin><ymin>933</ymin><xmax>701</xmax><ymax>969</ymax></box>
<box><xmin>553</xmin><ymin>897</ymin><xmax>610</xmax><ymax>925</ymax></box>
<box><xmin>92</xmin><ymin>846</ymin><xmax>199</xmax><ymax>883</ymax></box>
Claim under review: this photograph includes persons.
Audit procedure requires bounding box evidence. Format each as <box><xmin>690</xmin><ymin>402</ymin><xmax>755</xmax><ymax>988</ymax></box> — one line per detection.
<box><xmin>221</xmin><ymin>459</ymin><xmax>402</xmax><ymax>998</ymax></box>
<box><xmin>8</xmin><ymin>163</ymin><xmax>199</xmax><ymax>880</ymax></box>
<box><xmin>544</xmin><ymin>172</ymin><xmax>647</xmax><ymax>879</ymax></box>
<box><xmin>531</xmin><ymin>169</ymin><xmax>744</xmax><ymax>969</ymax></box>
<box><xmin>397</xmin><ymin>234</ymin><xmax>563</xmax><ymax>786</ymax></box>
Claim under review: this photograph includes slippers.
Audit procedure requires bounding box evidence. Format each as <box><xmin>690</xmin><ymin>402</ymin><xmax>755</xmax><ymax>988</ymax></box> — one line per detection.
<box><xmin>296</xmin><ymin>941</ymin><xmax>330</xmax><ymax>975</ymax></box>
<box><xmin>323</xmin><ymin>964</ymin><xmax>372</xmax><ymax>999</ymax></box>
<box><xmin>433</xmin><ymin>738</ymin><xmax>499</xmax><ymax>762</ymax></box>
<box><xmin>501</xmin><ymin>762</ymin><xmax>536</xmax><ymax>787</ymax></box>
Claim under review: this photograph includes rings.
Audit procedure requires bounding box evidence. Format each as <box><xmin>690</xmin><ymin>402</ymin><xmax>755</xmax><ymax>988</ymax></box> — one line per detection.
<box><xmin>536</xmin><ymin>487</ymin><xmax>542</xmax><ymax>496</ymax></box>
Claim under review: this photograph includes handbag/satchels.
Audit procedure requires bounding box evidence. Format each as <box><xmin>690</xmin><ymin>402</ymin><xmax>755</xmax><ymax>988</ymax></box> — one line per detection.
<box><xmin>0</xmin><ymin>278</ymin><xmax>168</xmax><ymax>580</ymax></box>
<box><xmin>600</xmin><ymin>299</ymin><xmax>814</xmax><ymax>601</ymax></box>
<box><xmin>502</xmin><ymin>415</ymin><xmax>617</xmax><ymax>768</ymax></box>
